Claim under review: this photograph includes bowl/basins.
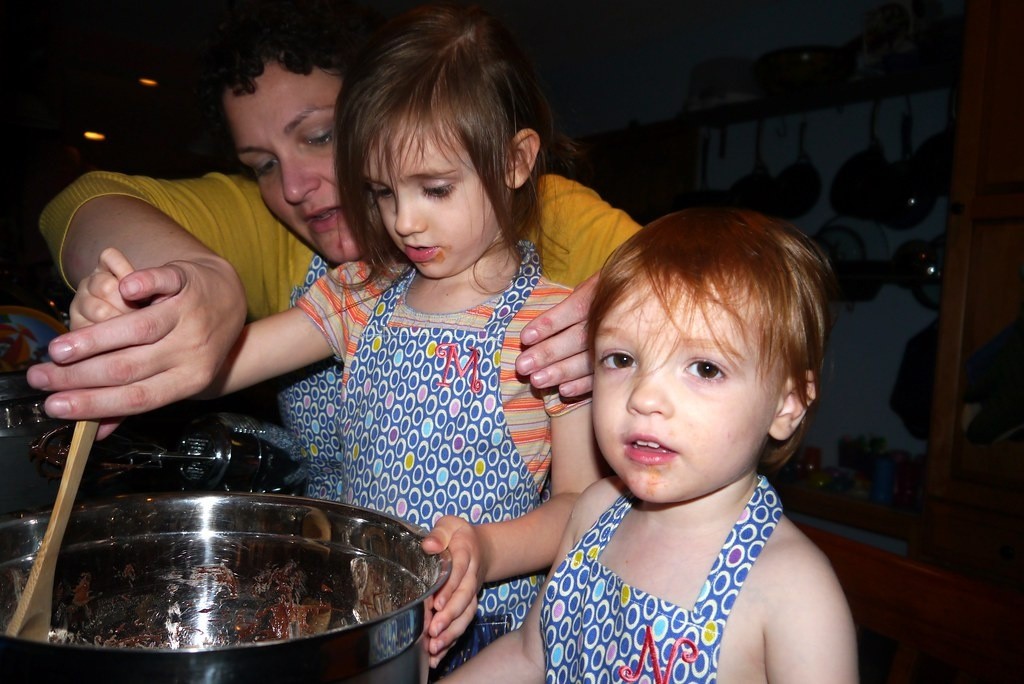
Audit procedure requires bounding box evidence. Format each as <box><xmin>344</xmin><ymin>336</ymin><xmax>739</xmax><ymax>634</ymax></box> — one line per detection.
<box><xmin>752</xmin><ymin>44</ymin><xmax>842</xmax><ymax>90</ymax></box>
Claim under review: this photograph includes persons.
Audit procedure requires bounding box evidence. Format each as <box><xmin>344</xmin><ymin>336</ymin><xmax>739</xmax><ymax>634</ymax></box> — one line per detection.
<box><xmin>26</xmin><ymin>0</ymin><xmax>643</xmax><ymax>440</ymax></box>
<box><xmin>72</xmin><ymin>0</ymin><xmax>609</xmax><ymax>684</ymax></box>
<box><xmin>425</xmin><ymin>207</ymin><xmax>858</xmax><ymax>684</ymax></box>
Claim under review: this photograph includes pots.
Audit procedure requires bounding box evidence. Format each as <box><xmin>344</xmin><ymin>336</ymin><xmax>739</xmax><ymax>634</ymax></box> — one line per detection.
<box><xmin>0</xmin><ymin>491</ymin><xmax>453</xmax><ymax>684</ymax></box>
